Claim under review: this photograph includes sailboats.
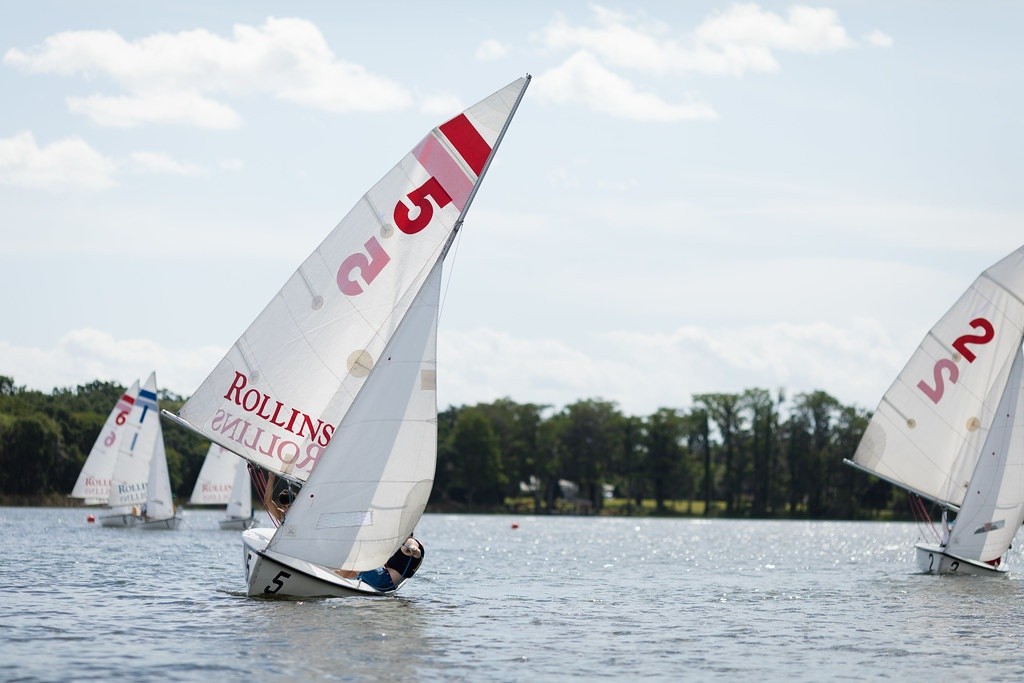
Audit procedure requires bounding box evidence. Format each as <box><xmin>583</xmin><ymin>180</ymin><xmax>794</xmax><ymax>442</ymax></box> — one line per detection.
<box><xmin>844</xmin><ymin>246</ymin><xmax>1023</xmax><ymax>578</ymax></box>
<box><xmin>65</xmin><ymin>372</ymin><xmax>176</xmax><ymax>532</ymax></box>
<box><xmin>150</xmin><ymin>71</ymin><xmax>531</xmax><ymax>601</ymax></box>
<box><xmin>186</xmin><ymin>438</ymin><xmax>266</xmax><ymax>533</ymax></box>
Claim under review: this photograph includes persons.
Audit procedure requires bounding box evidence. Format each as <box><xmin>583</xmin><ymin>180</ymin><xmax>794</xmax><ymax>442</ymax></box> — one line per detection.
<box><xmin>265</xmin><ymin>472</ymin><xmax>299</xmax><ymax>526</ymax></box>
<box><xmin>974</xmin><ymin>522</ymin><xmax>1012</xmax><ymax>566</ymax></box>
<box><xmin>357</xmin><ymin>531</ymin><xmax>425</xmax><ymax>592</ymax></box>
